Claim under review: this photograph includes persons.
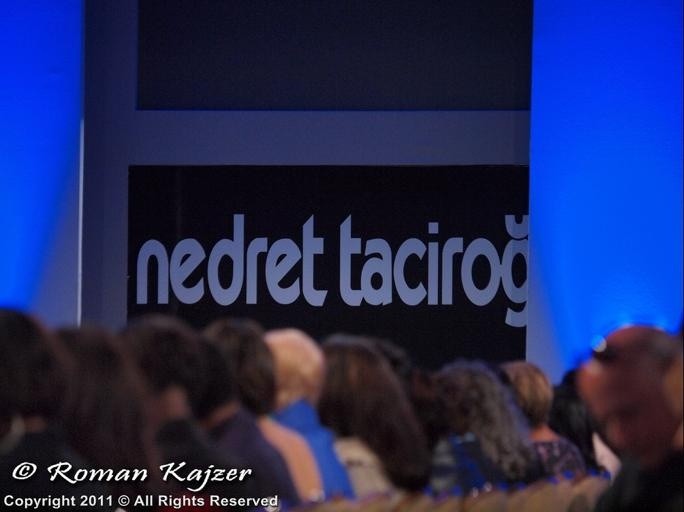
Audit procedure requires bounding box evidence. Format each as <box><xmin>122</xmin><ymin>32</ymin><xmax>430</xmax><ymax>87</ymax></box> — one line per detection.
<box><xmin>1</xmin><ymin>304</ymin><xmax>684</xmax><ymax>512</ymax></box>
